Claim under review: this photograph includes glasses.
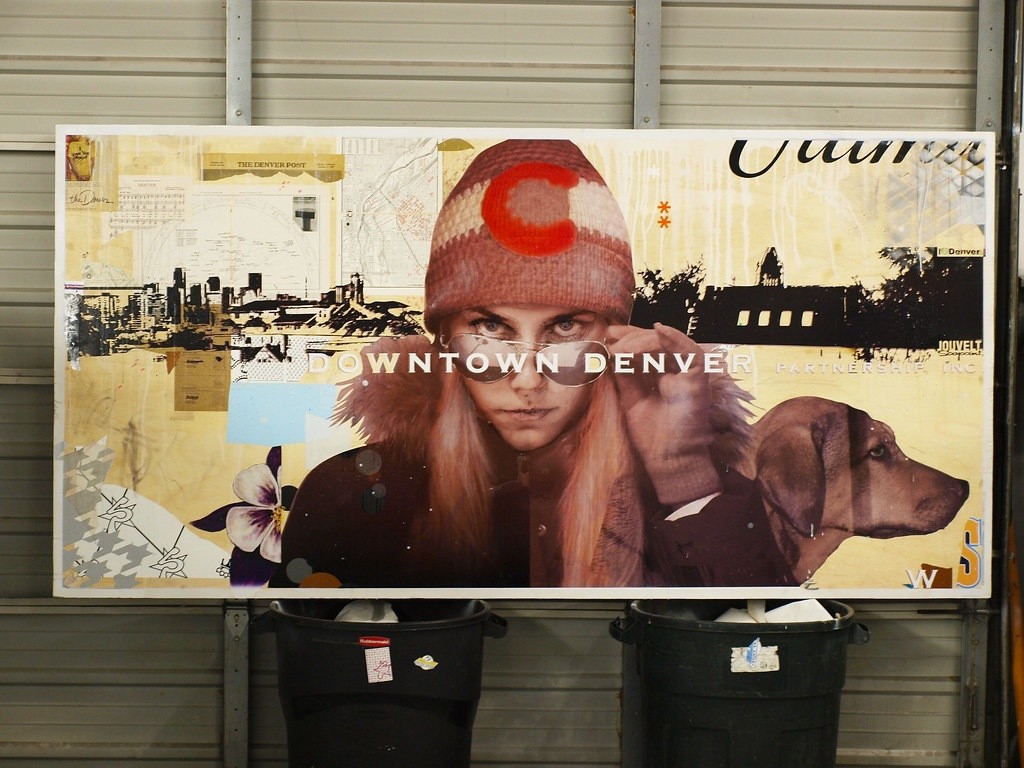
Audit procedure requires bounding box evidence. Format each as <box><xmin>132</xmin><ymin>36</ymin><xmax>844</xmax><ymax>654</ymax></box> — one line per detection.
<box><xmin>437</xmin><ymin>331</ymin><xmax>615</xmax><ymax>388</ymax></box>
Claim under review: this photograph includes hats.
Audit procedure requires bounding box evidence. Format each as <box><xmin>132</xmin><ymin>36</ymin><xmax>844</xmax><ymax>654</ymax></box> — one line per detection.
<box><xmin>424</xmin><ymin>137</ymin><xmax>635</xmax><ymax>327</ymax></box>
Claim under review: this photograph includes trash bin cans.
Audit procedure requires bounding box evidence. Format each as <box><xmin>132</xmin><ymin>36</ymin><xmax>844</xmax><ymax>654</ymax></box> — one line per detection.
<box><xmin>608</xmin><ymin>595</ymin><xmax>869</xmax><ymax>768</ymax></box>
<box><xmin>248</xmin><ymin>598</ymin><xmax>510</xmax><ymax>768</ymax></box>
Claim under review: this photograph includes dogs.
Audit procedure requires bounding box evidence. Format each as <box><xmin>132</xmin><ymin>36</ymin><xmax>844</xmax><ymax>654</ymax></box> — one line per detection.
<box><xmin>739</xmin><ymin>396</ymin><xmax>970</xmax><ymax>586</ymax></box>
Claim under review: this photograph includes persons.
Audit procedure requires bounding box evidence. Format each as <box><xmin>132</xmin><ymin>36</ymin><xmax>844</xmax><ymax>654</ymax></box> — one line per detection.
<box><xmin>279</xmin><ymin>137</ymin><xmax>795</xmax><ymax>588</ymax></box>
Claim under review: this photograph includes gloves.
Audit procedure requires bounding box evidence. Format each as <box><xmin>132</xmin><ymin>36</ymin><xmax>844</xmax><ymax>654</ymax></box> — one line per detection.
<box><xmin>603</xmin><ymin>323</ymin><xmax>723</xmax><ymax>507</ymax></box>
